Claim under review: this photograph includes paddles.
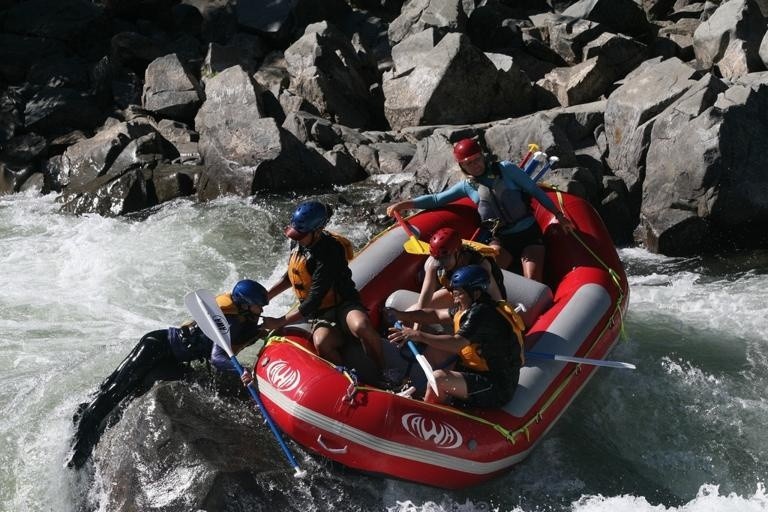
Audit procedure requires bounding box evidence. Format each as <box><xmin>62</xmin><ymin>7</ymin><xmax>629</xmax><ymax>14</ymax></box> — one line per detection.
<box><xmin>394</xmin><ymin>320</ymin><xmax>439</xmax><ymax>395</ymax></box>
<box><xmin>394</xmin><ymin>210</ymin><xmax>430</xmax><ymax>257</ymax></box>
<box><xmin>518</xmin><ymin>350</ymin><xmax>635</xmax><ymax>369</ymax></box>
<box><xmin>183</xmin><ymin>289</ymin><xmax>308</xmax><ymax>479</ymax></box>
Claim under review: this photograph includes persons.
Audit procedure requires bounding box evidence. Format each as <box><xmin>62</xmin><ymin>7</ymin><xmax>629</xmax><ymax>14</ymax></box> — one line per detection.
<box><xmin>383</xmin><ymin>265</ymin><xmax>526</xmax><ymax>410</ymax></box>
<box><xmin>386</xmin><ymin>139</ymin><xmax>575</xmax><ymax>283</ymax></box>
<box><xmin>405</xmin><ymin>228</ymin><xmax>506</xmax><ymax>326</ymax></box>
<box><xmin>67</xmin><ymin>280</ymin><xmax>268</xmax><ymax>469</ymax></box>
<box><xmin>261</xmin><ymin>202</ymin><xmax>384</xmax><ymax>369</ymax></box>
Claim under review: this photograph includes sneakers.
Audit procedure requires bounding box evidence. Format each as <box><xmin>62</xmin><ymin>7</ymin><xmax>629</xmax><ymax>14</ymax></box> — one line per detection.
<box><xmin>77</xmin><ymin>432</ymin><xmax>93</xmax><ymax>456</ymax></box>
<box><xmin>376</xmin><ymin>370</ymin><xmax>403</xmax><ymax>390</ymax></box>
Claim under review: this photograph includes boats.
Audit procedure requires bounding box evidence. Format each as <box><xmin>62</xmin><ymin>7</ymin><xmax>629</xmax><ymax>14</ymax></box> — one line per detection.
<box><xmin>251</xmin><ymin>185</ymin><xmax>630</xmax><ymax>491</ymax></box>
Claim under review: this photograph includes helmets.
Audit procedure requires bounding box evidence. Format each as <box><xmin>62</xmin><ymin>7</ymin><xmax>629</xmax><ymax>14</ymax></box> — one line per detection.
<box><xmin>453</xmin><ymin>137</ymin><xmax>482</xmax><ymax>165</ymax></box>
<box><xmin>450</xmin><ymin>263</ymin><xmax>491</xmax><ymax>300</ymax></box>
<box><xmin>232</xmin><ymin>277</ymin><xmax>272</xmax><ymax>309</ymax></box>
<box><xmin>284</xmin><ymin>201</ymin><xmax>334</xmax><ymax>240</ymax></box>
<box><xmin>429</xmin><ymin>227</ymin><xmax>463</xmax><ymax>260</ymax></box>
